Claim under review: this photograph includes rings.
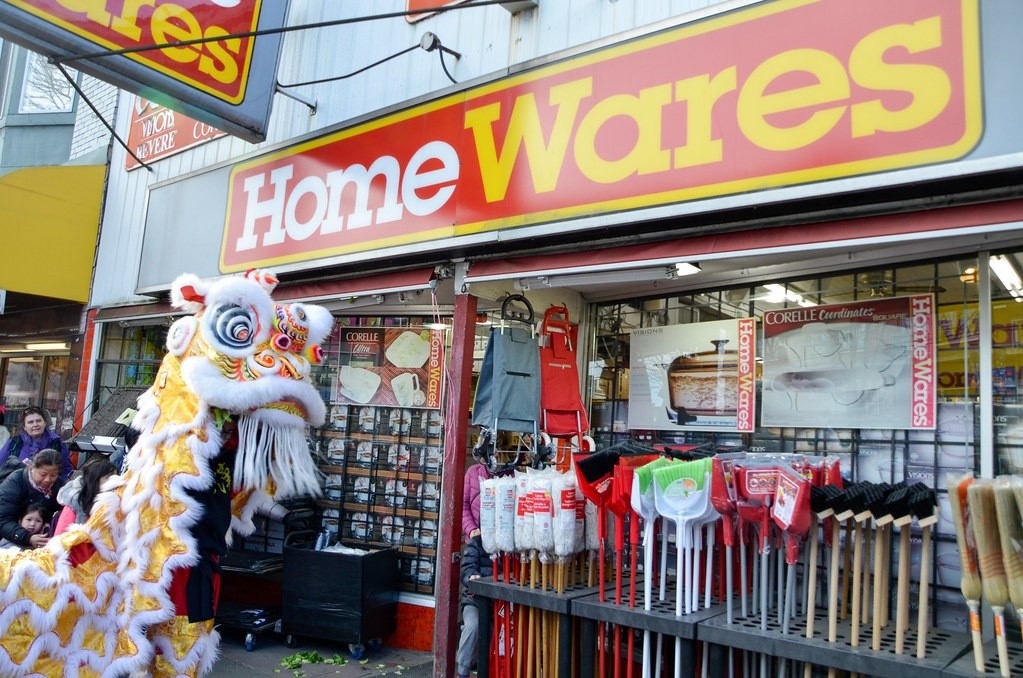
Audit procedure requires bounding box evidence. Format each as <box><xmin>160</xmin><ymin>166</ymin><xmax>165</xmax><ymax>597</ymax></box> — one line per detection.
<box><xmin>37</xmin><ymin>544</ymin><xmax>41</xmax><ymax>548</ymax></box>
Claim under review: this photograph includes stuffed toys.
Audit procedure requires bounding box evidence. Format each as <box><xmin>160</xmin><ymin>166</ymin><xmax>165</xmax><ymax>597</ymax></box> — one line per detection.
<box><xmin>0</xmin><ymin>269</ymin><xmax>336</xmax><ymax>678</ymax></box>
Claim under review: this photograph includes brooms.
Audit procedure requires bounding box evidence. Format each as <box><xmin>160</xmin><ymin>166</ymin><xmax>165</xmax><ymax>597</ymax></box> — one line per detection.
<box><xmin>575</xmin><ymin>441</ymin><xmax>1022</xmax><ymax>677</ymax></box>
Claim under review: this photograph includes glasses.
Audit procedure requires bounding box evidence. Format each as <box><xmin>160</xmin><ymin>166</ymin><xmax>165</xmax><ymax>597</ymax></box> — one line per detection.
<box><xmin>23</xmin><ymin>406</ymin><xmax>42</xmax><ymax>415</ymax></box>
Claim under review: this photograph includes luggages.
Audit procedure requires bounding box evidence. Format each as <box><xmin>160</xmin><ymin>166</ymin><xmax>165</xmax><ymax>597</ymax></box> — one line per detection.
<box><xmin>471</xmin><ymin>294</ymin><xmax>595</xmax><ymax>473</ymax></box>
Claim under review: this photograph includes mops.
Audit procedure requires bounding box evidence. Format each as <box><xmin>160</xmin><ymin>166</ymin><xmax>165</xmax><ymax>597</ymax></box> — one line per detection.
<box><xmin>475</xmin><ymin>464</ymin><xmax>599</xmax><ymax>677</ymax></box>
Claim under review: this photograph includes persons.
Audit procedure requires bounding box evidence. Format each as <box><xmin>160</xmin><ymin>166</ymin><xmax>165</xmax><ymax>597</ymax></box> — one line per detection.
<box><xmin>184</xmin><ymin>413</ymin><xmax>263</xmax><ymax>624</ymax></box>
<box><xmin>52</xmin><ymin>457</ymin><xmax>120</xmax><ymax>536</ymax></box>
<box><xmin>462</xmin><ymin>430</ymin><xmax>513</xmax><ymax>545</ymax></box>
<box><xmin>0</xmin><ymin>405</ymin><xmax>72</xmax><ymax>485</ymax></box>
<box><xmin>0</xmin><ymin>448</ymin><xmax>66</xmax><ymax>550</ymax></box>
<box><xmin>109</xmin><ymin>426</ymin><xmax>141</xmax><ymax>476</ymax></box>
<box><xmin>0</xmin><ymin>502</ymin><xmax>46</xmax><ymax>550</ymax></box>
<box><xmin>456</xmin><ymin>514</ymin><xmax>504</xmax><ymax>678</ymax></box>
<box><xmin>0</xmin><ymin>410</ymin><xmax>11</xmax><ymax>455</ymax></box>
